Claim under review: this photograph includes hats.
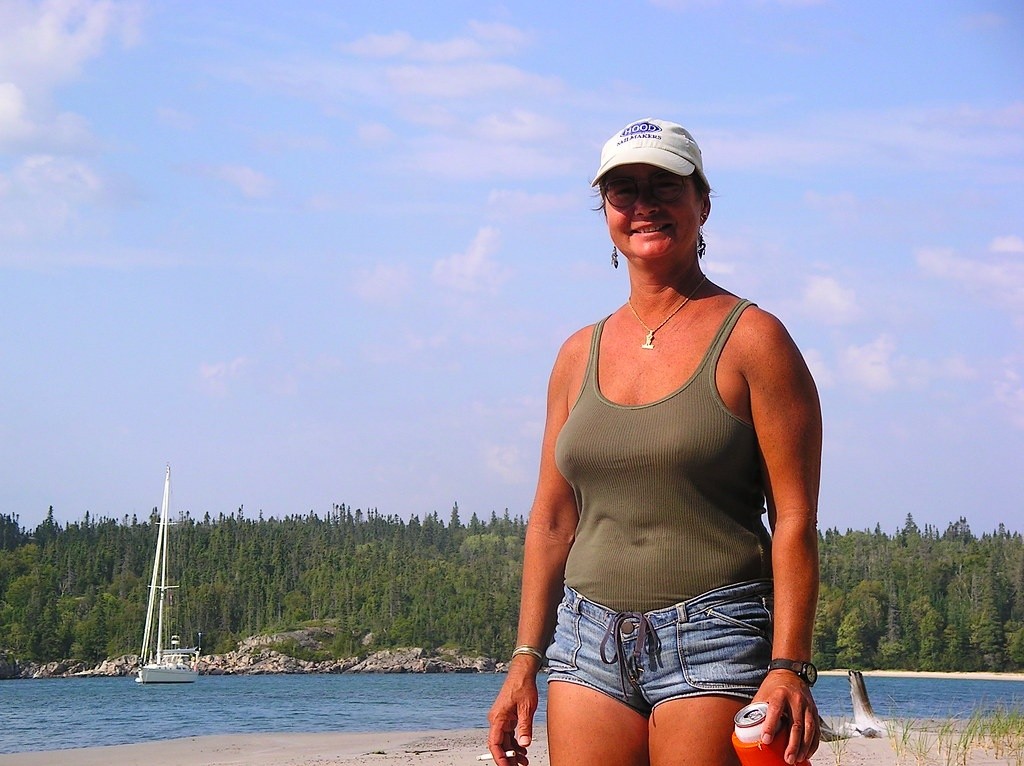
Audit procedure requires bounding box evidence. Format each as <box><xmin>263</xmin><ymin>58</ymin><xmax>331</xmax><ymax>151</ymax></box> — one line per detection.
<box><xmin>591</xmin><ymin>118</ymin><xmax>710</xmax><ymax>189</ymax></box>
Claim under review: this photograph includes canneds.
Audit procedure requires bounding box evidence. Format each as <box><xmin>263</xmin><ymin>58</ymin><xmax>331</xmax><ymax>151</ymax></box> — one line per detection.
<box><xmin>734</xmin><ymin>702</ymin><xmax>783</xmax><ymax>743</ymax></box>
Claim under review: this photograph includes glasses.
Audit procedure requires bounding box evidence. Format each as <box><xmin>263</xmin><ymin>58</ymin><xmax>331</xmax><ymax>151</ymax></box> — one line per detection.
<box><xmin>604</xmin><ymin>172</ymin><xmax>693</xmax><ymax>208</ymax></box>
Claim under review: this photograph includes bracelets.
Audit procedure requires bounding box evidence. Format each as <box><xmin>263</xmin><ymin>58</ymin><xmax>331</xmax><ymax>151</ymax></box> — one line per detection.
<box><xmin>512</xmin><ymin>645</ymin><xmax>543</xmax><ymax>671</ymax></box>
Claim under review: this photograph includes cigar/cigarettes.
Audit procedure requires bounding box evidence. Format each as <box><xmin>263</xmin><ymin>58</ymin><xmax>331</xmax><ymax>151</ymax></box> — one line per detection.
<box><xmin>475</xmin><ymin>751</ymin><xmax>515</xmax><ymax>761</ymax></box>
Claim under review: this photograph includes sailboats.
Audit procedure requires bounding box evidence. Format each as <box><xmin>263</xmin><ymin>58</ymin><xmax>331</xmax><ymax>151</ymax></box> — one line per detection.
<box><xmin>137</xmin><ymin>465</ymin><xmax>200</xmax><ymax>684</ymax></box>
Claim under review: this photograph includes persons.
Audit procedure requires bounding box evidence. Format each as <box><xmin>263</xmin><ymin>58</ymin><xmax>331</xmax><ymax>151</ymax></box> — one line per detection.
<box><xmin>489</xmin><ymin>119</ymin><xmax>824</xmax><ymax>766</ymax></box>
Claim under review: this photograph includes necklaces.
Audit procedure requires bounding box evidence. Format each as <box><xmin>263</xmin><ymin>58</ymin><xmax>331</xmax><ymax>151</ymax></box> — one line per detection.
<box><xmin>626</xmin><ymin>274</ymin><xmax>705</xmax><ymax>350</ymax></box>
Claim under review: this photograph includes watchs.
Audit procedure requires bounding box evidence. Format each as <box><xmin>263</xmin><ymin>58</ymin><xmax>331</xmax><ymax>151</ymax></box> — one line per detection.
<box><xmin>768</xmin><ymin>657</ymin><xmax>818</xmax><ymax>688</ymax></box>
